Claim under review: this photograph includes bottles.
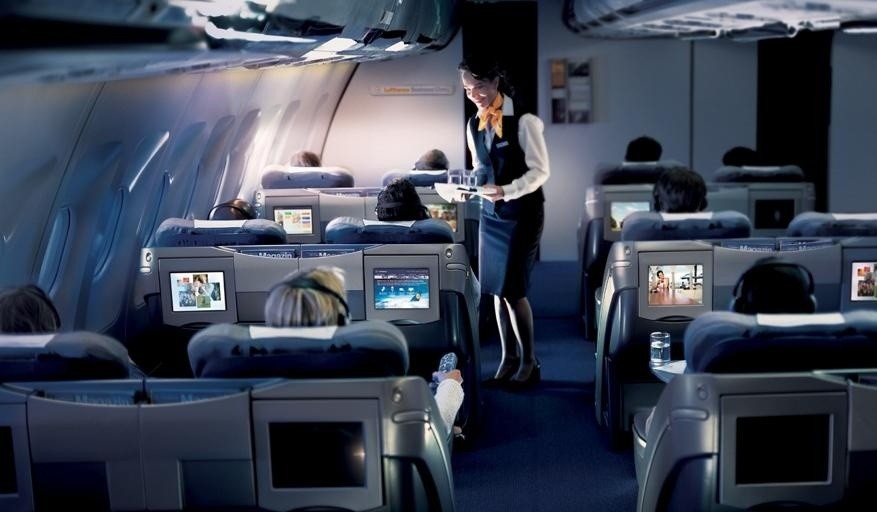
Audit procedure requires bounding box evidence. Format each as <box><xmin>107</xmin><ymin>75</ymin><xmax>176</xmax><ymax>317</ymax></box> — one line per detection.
<box><xmin>437</xmin><ymin>351</ymin><xmax>458</xmax><ymax>374</ymax></box>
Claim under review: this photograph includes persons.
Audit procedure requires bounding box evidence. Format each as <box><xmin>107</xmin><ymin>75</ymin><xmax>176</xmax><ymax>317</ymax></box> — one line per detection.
<box><xmin>293</xmin><ymin>150</ymin><xmax>321</xmax><ymax>171</ymax></box>
<box><xmin>264</xmin><ymin>263</ymin><xmax>354</xmax><ymax>327</ymax></box>
<box><xmin>201</xmin><ymin>199</ymin><xmax>257</xmax><ymax>222</ymax></box>
<box><xmin>457</xmin><ymin>51</ymin><xmax>552</xmax><ymax>389</ymax></box>
<box><xmin>653</xmin><ymin>271</ymin><xmax>671</xmax><ymax>290</ymax></box>
<box><xmin>650</xmin><ymin>164</ymin><xmax>710</xmax><ymax>216</ymax></box>
<box><xmin>373</xmin><ymin>176</ymin><xmax>431</xmax><ymax>225</ymax></box>
<box><xmin>439</xmin><ymin>206</ymin><xmax>457</xmax><ymax>233</ymax></box>
<box><xmin>412</xmin><ymin>144</ymin><xmax>451</xmax><ymax>173</ymax></box>
<box><xmin>431</xmin><ymin>205</ymin><xmax>439</xmax><ymax>219</ymax></box>
<box><xmin>721</xmin><ymin>146</ymin><xmax>764</xmax><ymax>169</ymax></box>
<box><xmin>2</xmin><ymin>280</ymin><xmax>65</xmax><ymax>335</ymax></box>
<box><xmin>724</xmin><ymin>252</ymin><xmax>819</xmax><ymax>319</ymax></box>
<box><xmin>857</xmin><ymin>264</ymin><xmax>877</xmax><ymax>297</ymax></box>
<box><xmin>176</xmin><ymin>274</ymin><xmax>220</xmax><ymax>309</ymax></box>
<box><xmin>624</xmin><ymin>134</ymin><xmax>665</xmax><ymax>164</ymax></box>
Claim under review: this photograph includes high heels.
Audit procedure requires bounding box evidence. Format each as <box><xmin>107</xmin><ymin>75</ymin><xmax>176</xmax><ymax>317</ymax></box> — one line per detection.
<box><xmin>485</xmin><ymin>360</ymin><xmax>541</xmax><ymax>388</ymax></box>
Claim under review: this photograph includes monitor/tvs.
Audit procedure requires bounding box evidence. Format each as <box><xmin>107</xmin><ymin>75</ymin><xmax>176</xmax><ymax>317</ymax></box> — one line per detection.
<box><xmin>251</xmin><ymin>398</ymin><xmax>384</xmax><ymax>512</ymax></box>
<box><xmin>638</xmin><ymin>250</ymin><xmax>714</xmax><ymax>321</ymax></box>
<box><xmin>363</xmin><ymin>256</ymin><xmax>441</xmax><ymax>325</ymax></box>
<box><xmin>159</xmin><ymin>257</ymin><xmax>239</xmax><ymax>327</ymax></box>
<box><xmin>603</xmin><ymin>191</ymin><xmax>657</xmax><ymax>241</ymax></box>
<box><xmin>720</xmin><ymin>391</ymin><xmax>848</xmax><ymax>510</ymax></box>
<box><xmin>841</xmin><ymin>248</ymin><xmax>877</xmax><ymax>312</ymax></box>
<box><xmin>0</xmin><ymin>403</ymin><xmax>36</xmax><ymax>512</ymax></box>
<box><xmin>748</xmin><ymin>189</ymin><xmax>802</xmax><ymax>236</ymax></box>
<box><xmin>265</xmin><ymin>195</ymin><xmax>322</xmax><ymax>243</ymax></box>
<box><xmin>419</xmin><ymin>194</ymin><xmax>465</xmax><ymax>242</ymax></box>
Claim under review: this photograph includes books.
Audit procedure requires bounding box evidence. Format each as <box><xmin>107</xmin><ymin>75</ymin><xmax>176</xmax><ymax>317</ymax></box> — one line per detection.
<box><xmin>433</xmin><ymin>182</ymin><xmax>497</xmax><ymax>204</ymax></box>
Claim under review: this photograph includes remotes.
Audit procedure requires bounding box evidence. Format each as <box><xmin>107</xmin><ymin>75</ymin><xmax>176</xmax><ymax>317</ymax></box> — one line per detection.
<box><xmin>438</xmin><ymin>353</ymin><xmax>457</xmax><ymax>372</ymax></box>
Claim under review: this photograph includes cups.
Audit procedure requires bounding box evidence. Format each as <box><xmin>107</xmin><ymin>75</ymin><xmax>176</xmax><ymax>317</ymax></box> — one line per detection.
<box><xmin>648</xmin><ymin>330</ymin><xmax>672</xmax><ymax>369</ymax></box>
<box><xmin>447</xmin><ymin>169</ymin><xmax>461</xmax><ymax>185</ymax></box>
<box><xmin>463</xmin><ymin>169</ymin><xmax>478</xmax><ymax>186</ymax></box>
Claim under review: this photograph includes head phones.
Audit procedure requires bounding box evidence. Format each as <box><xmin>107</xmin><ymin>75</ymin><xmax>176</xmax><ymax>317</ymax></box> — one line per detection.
<box><xmin>653</xmin><ymin>167</ymin><xmax>707</xmax><ymax>212</ymax></box>
<box><xmin>415</xmin><ymin>162</ymin><xmax>448</xmax><ymax>170</ymax></box>
<box><xmin>729</xmin><ymin>257</ymin><xmax>819</xmax><ymax>313</ymax></box>
<box><xmin>376</xmin><ymin>202</ymin><xmax>432</xmax><ymax>218</ymax></box>
<box><xmin>268</xmin><ymin>278</ymin><xmax>351</xmax><ymax>326</ymax></box>
<box><xmin>207</xmin><ymin>203</ymin><xmax>256</xmax><ymax>219</ymax></box>
